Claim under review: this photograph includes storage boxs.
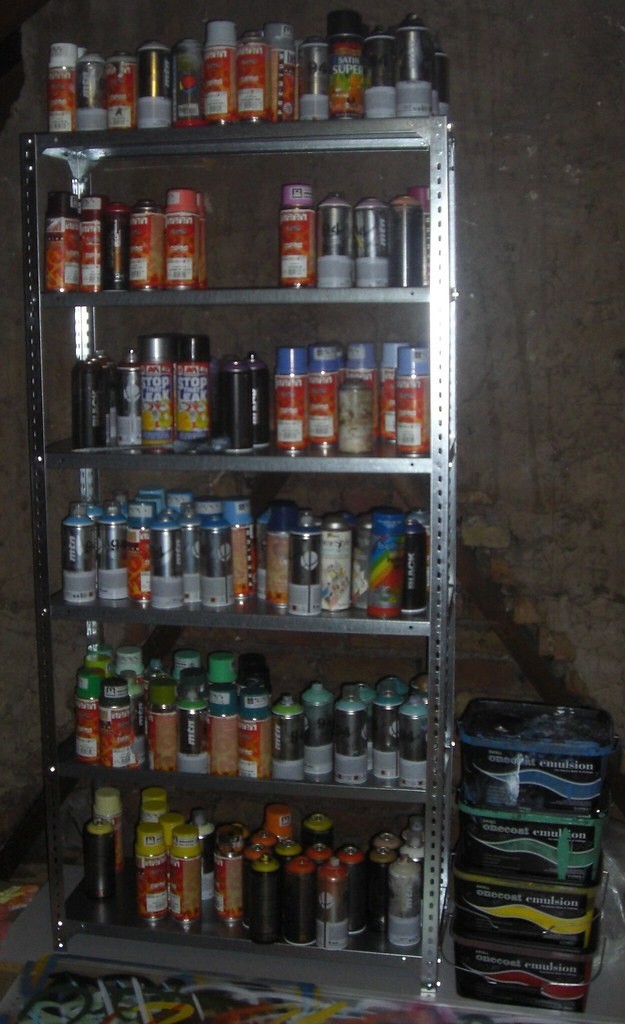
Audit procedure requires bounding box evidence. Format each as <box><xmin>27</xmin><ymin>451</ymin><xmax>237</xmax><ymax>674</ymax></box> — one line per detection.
<box><xmin>455</xmin><ymin>697</ymin><xmax>620</xmax><ymax>816</ymax></box>
<box><xmin>455</xmin><ymin>794</ymin><xmax>607</xmax><ymax>887</ymax></box>
<box><xmin>440</xmin><ymin>916</ymin><xmax>608</xmax><ymax>1014</ymax></box>
<box><xmin>451</xmin><ymin>853</ymin><xmax>611</xmax><ymax>953</ymax></box>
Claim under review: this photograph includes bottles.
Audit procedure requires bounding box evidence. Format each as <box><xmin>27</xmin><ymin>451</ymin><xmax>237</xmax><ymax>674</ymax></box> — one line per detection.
<box><xmin>45</xmin><ymin>189</ymin><xmax>207</xmax><ymax>291</ymax></box>
<box><xmin>395</xmin><ymin>347</ymin><xmax>431</xmax><ymax>457</ymax></box>
<box><xmin>370</xmin><ymin>815</ymin><xmax>424</xmax><ymax>946</ymax></box>
<box><xmin>85</xmin><ymin>819</ymin><xmax>114</xmax><ymax>900</ymax></box>
<box><xmin>135</xmin><ymin>787</ymin><xmax>214</xmax><ymax>923</ymax></box>
<box><xmin>93</xmin><ymin>788</ymin><xmax>123</xmax><ymax>873</ymax></box>
<box><xmin>334</xmin><ymin>674</ymin><xmax>429</xmax><ymax>788</ymax></box>
<box><xmin>256</xmin><ymin>505</ymin><xmax>321</xmax><ymax>616</ymax></box>
<box><xmin>215</xmin><ymin>803</ymin><xmax>366</xmax><ymax>949</ymax></box>
<box><xmin>61</xmin><ymin>487</ymin><xmax>255</xmax><ymax>609</ymax></box>
<box><xmin>321</xmin><ymin>508</ymin><xmax>431</xmax><ymax>618</ymax></box>
<box><xmin>76</xmin><ymin>334</ymin><xmax>269</xmax><ymax>453</ymax></box>
<box><xmin>275</xmin><ymin>342</ymin><xmax>378</xmax><ymax>452</ymax></box>
<box><xmin>302</xmin><ymin>684</ymin><xmax>333</xmax><ymax>774</ymax></box>
<box><xmin>272</xmin><ymin>695</ymin><xmax>304</xmax><ymax>781</ymax></box>
<box><xmin>238</xmin><ymin>13</ymin><xmax>449</xmax><ymax>125</ymax></box>
<box><xmin>49</xmin><ymin>20</ymin><xmax>237</xmax><ymax>132</ymax></box>
<box><xmin>75</xmin><ymin>646</ymin><xmax>271</xmax><ymax>779</ymax></box>
<box><xmin>380</xmin><ymin>343</ymin><xmax>409</xmax><ymax>442</ymax></box>
<box><xmin>280</xmin><ymin>185</ymin><xmax>430</xmax><ymax>288</ymax></box>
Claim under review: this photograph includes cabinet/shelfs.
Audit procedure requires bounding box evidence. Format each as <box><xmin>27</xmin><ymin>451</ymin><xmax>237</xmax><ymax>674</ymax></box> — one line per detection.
<box><xmin>19</xmin><ymin>118</ymin><xmax>458</xmax><ymax>995</ymax></box>
